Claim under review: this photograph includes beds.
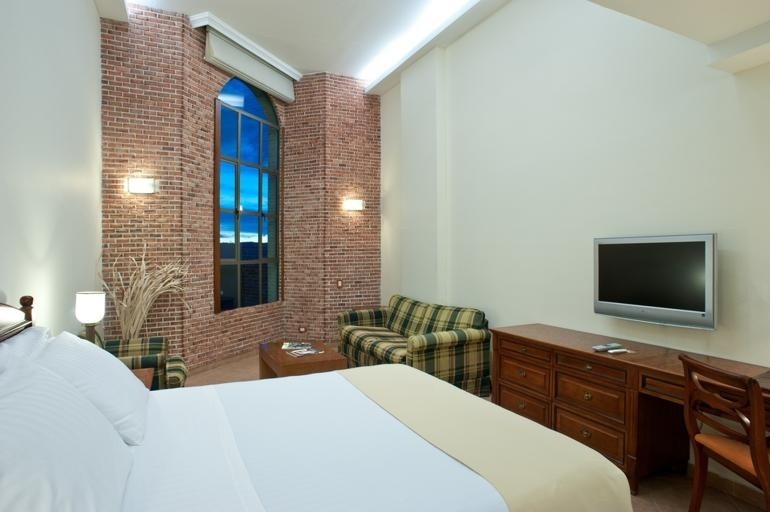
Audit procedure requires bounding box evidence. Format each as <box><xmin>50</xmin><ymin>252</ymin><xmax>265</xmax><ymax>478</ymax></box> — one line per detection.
<box><xmin>1</xmin><ymin>293</ymin><xmax>637</xmax><ymax>511</ymax></box>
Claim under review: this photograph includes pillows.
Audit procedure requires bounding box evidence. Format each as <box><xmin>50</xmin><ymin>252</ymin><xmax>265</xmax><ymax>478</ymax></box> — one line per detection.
<box><xmin>2</xmin><ymin>325</ymin><xmax>151</xmax><ymax>510</ymax></box>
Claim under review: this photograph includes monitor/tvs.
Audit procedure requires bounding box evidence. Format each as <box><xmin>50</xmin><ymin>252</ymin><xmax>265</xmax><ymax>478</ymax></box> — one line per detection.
<box><xmin>592</xmin><ymin>232</ymin><xmax>718</xmax><ymax>331</ymax></box>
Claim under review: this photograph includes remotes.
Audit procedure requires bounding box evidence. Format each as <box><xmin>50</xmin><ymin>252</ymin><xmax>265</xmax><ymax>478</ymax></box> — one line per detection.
<box><xmin>591</xmin><ymin>342</ymin><xmax>623</xmax><ymax>352</ymax></box>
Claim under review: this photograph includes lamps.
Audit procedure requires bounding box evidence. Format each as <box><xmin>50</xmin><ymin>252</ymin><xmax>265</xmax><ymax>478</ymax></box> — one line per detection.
<box><xmin>75</xmin><ymin>291</ymin><xmax>107</xmax><ymax>343</ymax></box>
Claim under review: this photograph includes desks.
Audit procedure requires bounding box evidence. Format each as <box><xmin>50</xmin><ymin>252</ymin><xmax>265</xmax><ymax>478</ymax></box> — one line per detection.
<box><xmin>630</xmin><ymin>346</ymin><xmax>770</xmax><ymax>495</ymax></box>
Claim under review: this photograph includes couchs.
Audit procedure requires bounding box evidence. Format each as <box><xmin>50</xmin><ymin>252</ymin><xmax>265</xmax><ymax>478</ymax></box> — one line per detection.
<box><xmin>335</xmin><ymin>294</ymin><xmax>496</xmax><ymax>398</ymax></box>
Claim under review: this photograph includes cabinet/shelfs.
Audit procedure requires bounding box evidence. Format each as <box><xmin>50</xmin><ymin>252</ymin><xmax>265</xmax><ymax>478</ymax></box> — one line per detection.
<box><xmin>490</xmin><ymin>322</ymin><xmax>682</xmax><ymax>497</ymax></box>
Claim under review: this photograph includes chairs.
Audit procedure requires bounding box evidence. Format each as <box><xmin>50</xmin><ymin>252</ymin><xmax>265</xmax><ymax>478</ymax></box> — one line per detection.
<box><xmin>79</xmin><ymin>328</ymin><xmax>188</xmax><ymax>390</ymax></box>
<box><xmin>678</xmin><ymin>352</ymin><xmax>768</xmax><ymax>511</ymax></box>
